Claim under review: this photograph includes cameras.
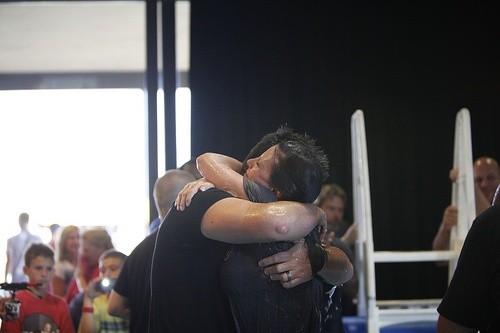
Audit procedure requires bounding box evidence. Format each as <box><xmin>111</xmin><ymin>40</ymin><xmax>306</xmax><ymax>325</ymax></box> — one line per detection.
<box><xmin>4</xmin><ymin>301</ymin><xmax>21</xmax><ymax>319</ymax></box>
<box><xmin>94</xmin><ymin>278</ymin><xmax>118</xmax><ymax>294</ymax></box>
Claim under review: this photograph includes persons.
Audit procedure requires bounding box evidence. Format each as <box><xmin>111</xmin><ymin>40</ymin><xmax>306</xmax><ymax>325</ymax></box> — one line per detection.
<box><xmin>105</xmin><ymin>128</ymin><xmax>358</xmax><ymax>333</ymax></box>
<box><xmin>0</xmin><ymin>213</ymin><xmax>129</xmax><ymax>333</ymax></box>
<box><xmin>436</xmin><ymin>206</ymin><xmax>500</xmax><ymax>333</ymax></box>
<box><xmin>432</xmin><ymin>157</ymin><xmax>500</xmax><ymax>269</ymax></box>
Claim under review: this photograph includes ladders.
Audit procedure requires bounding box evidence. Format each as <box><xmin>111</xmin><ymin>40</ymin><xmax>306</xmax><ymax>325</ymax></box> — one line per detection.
<box><xmin>343</xmin><ymin>109</ymin><xmax>477</xmax><ymax>330</ymax></box>
